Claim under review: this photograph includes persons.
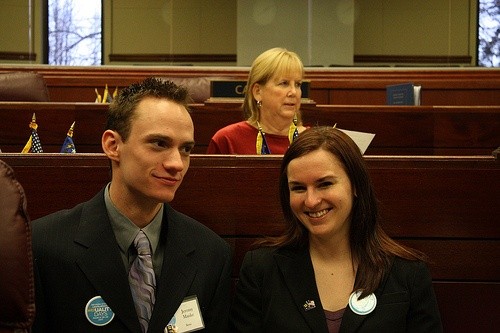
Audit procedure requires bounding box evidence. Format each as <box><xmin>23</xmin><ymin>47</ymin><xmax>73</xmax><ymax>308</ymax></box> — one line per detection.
<box><xmin>236</xmin><ymin>127</ymin><xmax>444</xmax><ymax>333</ymax></box>
<box><xmin>205</xmin><ymin>47</ymin><xmax>309</xmax><ymax>154</ymax></box>
<box><xmin>30</xmin><ymin>77</ymin><xmax>236</xmax><ymax>333</ymax></box>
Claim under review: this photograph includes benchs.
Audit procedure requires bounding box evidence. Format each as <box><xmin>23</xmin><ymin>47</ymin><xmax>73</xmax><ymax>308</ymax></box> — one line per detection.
<box><xmin>0</xmin><ymin>101</ymin><xmax>500</xmax><ymax>153</ymax></box>
<box><xmin>0</xmin><ymin>154</ymin><xmax>500</xmax><ymax>333</ymax></box>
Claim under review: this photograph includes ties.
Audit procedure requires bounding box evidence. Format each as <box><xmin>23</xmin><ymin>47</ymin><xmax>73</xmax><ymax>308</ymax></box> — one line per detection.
<box><xmin>128</xmin><ymin>231</ymin><xmax>157</xmax><ymax>333</ymax></box>
